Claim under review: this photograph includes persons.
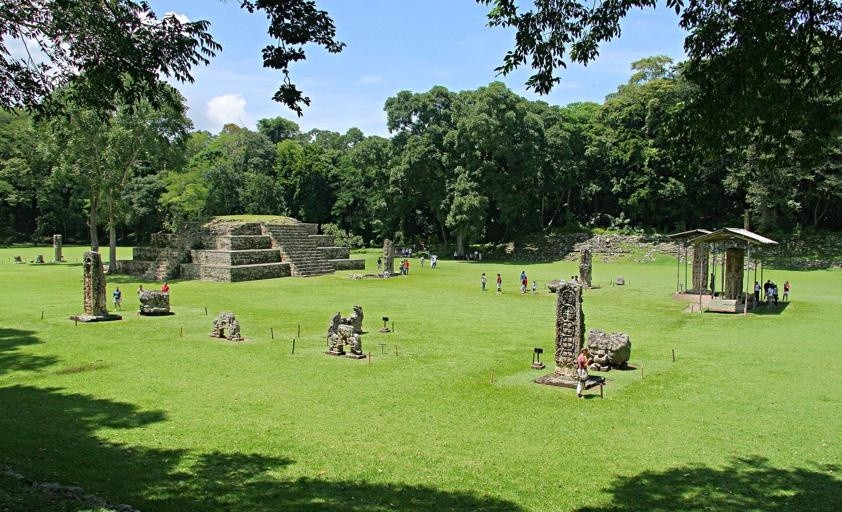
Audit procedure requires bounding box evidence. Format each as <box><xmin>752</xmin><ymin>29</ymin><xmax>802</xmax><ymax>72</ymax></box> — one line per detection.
<box><xmin>376</xmin><ymin>247</ymin><xmax>438</xmax><ymax>276</ymax></box>
<box><xmin>111</xmin><ymin>286</ymin><xmax>123</xmax><ymax>311</ymax></box>
<box><xmin>710</xmin><ymin>273</ymin><xmax>715</xmax><ymax>295</ymax></box>
<box><xmin>136</xmin><ymin>284</ymin><xmax>145</xmax><ymax>295</ymax></box>
<box><xmin>522</xmin><ymin>277</ymin><xmax>528</xmax><ymax>292</ymax></box>
<box><xmin>160</xmin><ymin>281</ymin><xmax>170</xmax><ymax>294</ymax></box>
<box><xmin>520</xmin><ymin>285</ymin><xmax>526</xmax><ymax>294</ymax></box>
<box><xmin>575</xmin><ymin>347</ymin><xmax>593</xmax><ymax>400</ymax></box>
<box><xmin>480</xmin><ymin>272</ymin><xmax>487</xmax><ymax>291</ymax></box>
<box><xmin>754</xmin><ymin>279</ymin><xmax>792</xmax><ymax>307</ymax></box>
<box><xmin>520</xmin><ymin>271</ymin><xmax>526</xmax><ymax>284</ymax></box>
<box><xmin>532</xmin><ymin>280</ymin><xmax>538</xmax><ymax>293</ymax></box>
<box><xmin>496</xmin><ymin>273</ymin><xmax>504</xmax><ymax>294</ymax></box>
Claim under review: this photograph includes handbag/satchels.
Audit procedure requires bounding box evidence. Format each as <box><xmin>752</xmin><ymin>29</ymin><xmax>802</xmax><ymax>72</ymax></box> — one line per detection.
<box><xmin>578</xmin><ymin>369</ymin><xmax>588</xmax><ymax>381</ymax></box>
